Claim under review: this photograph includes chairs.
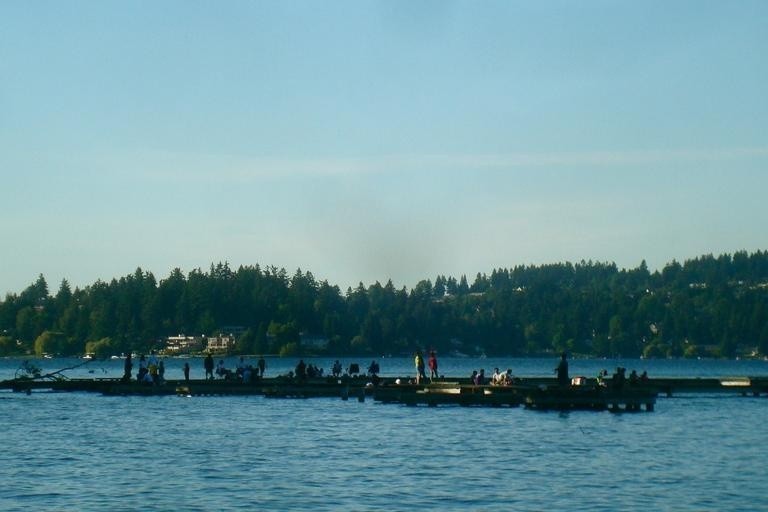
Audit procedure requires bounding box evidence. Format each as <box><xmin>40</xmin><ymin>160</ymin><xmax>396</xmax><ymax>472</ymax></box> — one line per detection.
<box><xmin>368</xmin><ymin>363</ymin><xmax>379</xmax><ymax>377</ymax></box>
<box><xmin>332</xmin><ymin>363</ymin><xmax>342</xmax><ymax>376</ymax></box>
<box><xmin>216</xmin><ymin>364</ymin><xmax>225</xmax><ymax>380</ymax></box>
<box><xmin>350</xmin><ymin>364</ymin><xmax>359</xmax><ymax>377</ymax></box>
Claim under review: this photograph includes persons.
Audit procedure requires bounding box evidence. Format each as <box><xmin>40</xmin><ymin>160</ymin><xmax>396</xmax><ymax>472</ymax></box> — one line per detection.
<box><xmin>333</xmin><ymin>360</ymin><xmax>342</xmax><ymax>378</ymax></box>
<box><xmin>415</xmin><ymin>352</ymin><xmax>428</xmax><ymax>384</ymax></box>
<box><xmin>368</xmin><ymin>361</ymin><xmax>380</xmax><ymax>377</ymax></box>
<box><xmin>557</xmin><ymin>352</ymin><xmax>568</xmax><ymax>385</ymax></box>
<box><xmin>428</xmin><ymin>351</ymin><xmax>438</xmax><ymax>383</ymax></box>
<box><xmin>596</xmin><ymin>367</ymin><xmax>649</xmax><ymax>393</ymax></box>
<box><xmin>287</xmin><ymin>359</ymin><xmax>323</xmax><ymax>384</ymax></box>
<box><xmin>182</xmin><ymin>363</ymin><xmax>189</xmax><ymax>383</ymax></box>
<box><xmin>204</xmin><ymin>354</ymin><xmax>267</xmax><ymax>383</ymax></box>
<box><xmin>471</xmin><ymin>368</ymin><xmax>513</xmax><ymax>385</ymax></box>
<box><xmin>120</xmin><ymin>350</ymin><xmax>167</xmax><ymax>385</ymax></box>
